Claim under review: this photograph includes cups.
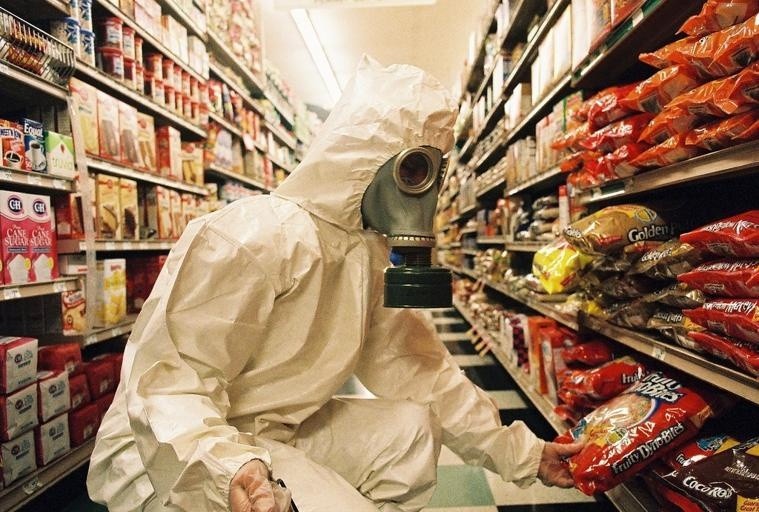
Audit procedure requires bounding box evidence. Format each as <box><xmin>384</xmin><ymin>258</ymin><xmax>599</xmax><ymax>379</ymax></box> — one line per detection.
<box><xmin>5</xmin><ymin>152</ymin><xmax>24</xmax><ymax>167</ymax></box>
<box><xmin>29</xmin><ymin>141</ymin><xmax>43</xmax><ymax>165</ymax></box>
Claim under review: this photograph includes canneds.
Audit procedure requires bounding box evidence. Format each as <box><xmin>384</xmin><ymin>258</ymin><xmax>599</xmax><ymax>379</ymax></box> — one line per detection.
<box><xmin>53</xmin><ymin>0</ymin><xmax>95</xmax><ymax>68</ymax></box>
<box><xmin>100</xmin><ymin>17</ymin><xmax>199</xmax><ymax>125</ymax></box>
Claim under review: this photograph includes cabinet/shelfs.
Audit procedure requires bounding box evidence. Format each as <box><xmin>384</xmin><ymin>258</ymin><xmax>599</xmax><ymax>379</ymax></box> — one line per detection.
<box><xmin>0</xmin><ymin>0</ymin><xmax>318</xmax><ymax>512</ymax></box>
<box><xmin>435</xmin><ymin>0</ymin><xmax>759</xmax><ymax>510</ymax></box>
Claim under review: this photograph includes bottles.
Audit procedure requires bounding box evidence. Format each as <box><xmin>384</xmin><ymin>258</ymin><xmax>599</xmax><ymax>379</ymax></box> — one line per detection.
<box><xmin>70</xmin><ymin>0</ymin><xmax>78</xmax><ymax>18</ymax></box>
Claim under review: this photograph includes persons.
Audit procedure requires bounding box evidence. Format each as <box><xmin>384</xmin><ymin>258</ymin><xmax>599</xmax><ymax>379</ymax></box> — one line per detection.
<box><xmin>85</xmin><ymin>52</ymin><xmax>585</xmax><ymax>512</ymax></box>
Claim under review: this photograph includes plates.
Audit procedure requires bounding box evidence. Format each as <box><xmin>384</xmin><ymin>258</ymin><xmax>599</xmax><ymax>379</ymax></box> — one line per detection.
<box><xmin>24</xmin><ymin>149</ymin><xmax>46</xmax><ymax>170</ymax></box>
